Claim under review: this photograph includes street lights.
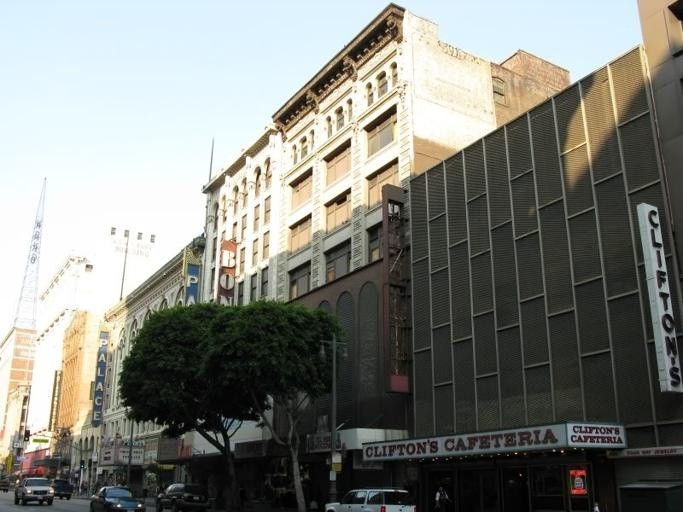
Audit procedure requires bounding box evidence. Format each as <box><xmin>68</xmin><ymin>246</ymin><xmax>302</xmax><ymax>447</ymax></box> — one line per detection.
<box><xmin>319</xmin><ymin>340</ymin><xmax>348</xmax><ymax>488</ymax></box>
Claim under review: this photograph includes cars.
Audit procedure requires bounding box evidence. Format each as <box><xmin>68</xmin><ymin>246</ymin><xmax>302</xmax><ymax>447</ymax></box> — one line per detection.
<box><xmin>90</xmin><ymin>486</ymin><xmax>146</xmax><ymax>512</ymax></box>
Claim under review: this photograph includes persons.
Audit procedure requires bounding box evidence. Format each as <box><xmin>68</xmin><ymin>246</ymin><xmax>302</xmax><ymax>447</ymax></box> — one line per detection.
<box><xmin>240</xmin><ymin>483</ymin><xmax>248</xmax><ymax>507</ymax></box>
<box><xmin>434</xmin><ymin>486</ymin><xmax>453</xmax><ymax>512</ymax></box>
<box><xmin>142</xmin><ymin>487</ymin><xmax>149</xmax><ymax>500</ymax></box>
<box><xmin>74</xmin><ymin>478</ymin><xmax>128</xmax><ymax>498</ymax></box>
<box><xmin>263</xmin><ymin>480</ymin><xmax>274</xmax><ymax>507</ymax></box>
<box><xmin>201</xmin><ymin>486</ymin><xmax>209</xmax><ymax>502</ymax></box>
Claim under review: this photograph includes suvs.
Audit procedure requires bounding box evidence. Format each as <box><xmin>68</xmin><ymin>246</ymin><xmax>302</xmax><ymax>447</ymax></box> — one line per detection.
<box><xmin>325</xmin><ymin>489</ymin><xmax>417</xmax><ymax>512</ymax></box>
<box><xmin>156</xmin><ymin>483</ymin><xmax>217</xmax><ymax>512</ymax></box>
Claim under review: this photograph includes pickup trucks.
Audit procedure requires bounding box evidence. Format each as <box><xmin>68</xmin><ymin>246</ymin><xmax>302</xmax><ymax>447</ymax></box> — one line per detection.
<box><xmin>15</xmin><ymin>478</ymin><xmax>54</xmax><ymax>505</ymax></box>
<box><xmin>51</xmin><ymin>480</ymin><xmax>73</xmax><ymax>500</ymax></box>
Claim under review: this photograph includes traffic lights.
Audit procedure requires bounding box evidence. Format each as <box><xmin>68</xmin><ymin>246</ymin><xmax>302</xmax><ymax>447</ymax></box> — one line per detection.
<box><xmin>80</xmin><ymin>459</ymin><xmax>85</xmax><ymax>469</ymax></box>
<box><xmin>23</xmin><ymin>431</ymin><xmax>30</xmax><ymax>441</ymax></box>
